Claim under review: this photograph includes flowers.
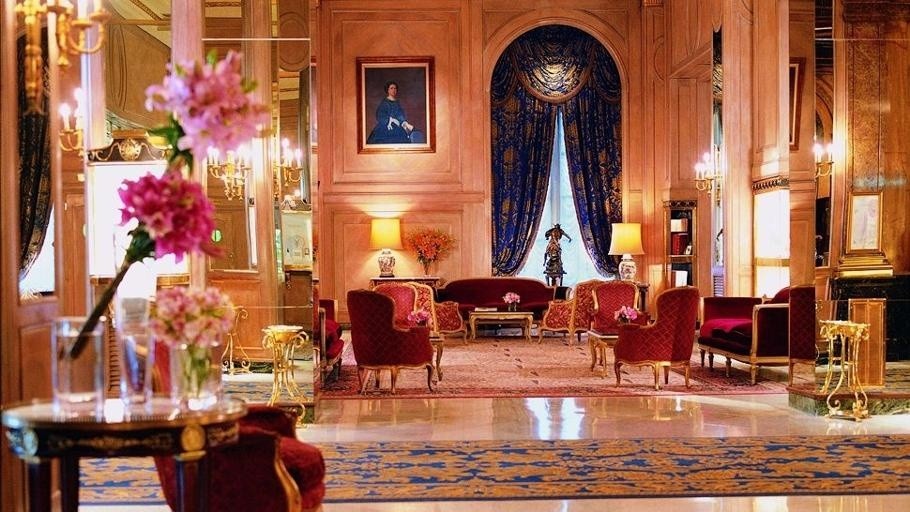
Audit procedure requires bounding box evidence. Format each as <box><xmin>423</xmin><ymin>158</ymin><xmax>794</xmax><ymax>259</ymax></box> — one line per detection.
<box><xmin>502</xmin><ymin>292</ymin><xmax>521</xmax><ymax>304</ymax></box>
<box><xmin>407</xmin><ymin>224</ymin><xmax>459</xmax><ymax>277</ymax></box>
<box><xmin>111</xmin><ymin>50</ymin><xmax>271</xmax><ymax>305</ymax></box>
<box><xmin>614</xmin><ymin>306</ymin><xmax>637</xmax><ymax>320</ymax></box>
<box><xmin>431</xmin><ymin>276</ymin><xmax>558</xmax><ymax>333</ymax></box>
<box><xmin>149</xmin><ymin>286</ymin><xmax>236</xmax><ymax>397</ymax></box>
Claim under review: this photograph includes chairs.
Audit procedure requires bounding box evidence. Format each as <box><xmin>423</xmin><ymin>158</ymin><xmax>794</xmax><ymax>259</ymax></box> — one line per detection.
<box><xmin>612</xmin><ymin>283</ymin><xmax>701</xmax><ymax>392</ymax></box>
<box><xmin>586</xmin><ymin>278</ymin><xmax>642</xmax><ymax>375</ymax></box>
<box><xmin>538</xmin><ymin>277</ymin><xmax>603</xmax><ymax>343</ymax></box>
<box><xmin>134</xmin><ymin>340</ymin><xmax>326</xmax><ymax>512</ymax></box>
<box><xmin>311</xmin><ymin>286</ymin><xmax>344</xmax><ymax>383</ymax></box>
<box><xmin>416</xmin><ymin>274</ymin><xmax>473</xmax><ymax>346</ymax></box>
<box><xmin>347</xmin><ymin>290</ymin><xmax>444</xmax><ymax>398</ymax></box>
<box><xmin>700</xmin><ymin>283</ymin><xmax>797</xmax><ymax>385</ymax></box>
<box><xmin>371</xmin><ymin>283</ymin><xmax>418</xmax><ymax>328</ymax></box>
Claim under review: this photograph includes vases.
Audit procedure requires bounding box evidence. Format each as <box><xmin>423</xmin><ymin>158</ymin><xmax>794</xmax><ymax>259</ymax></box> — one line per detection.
<box><xmin>168</xmin><ymin>342</ymin><xmax>218</xmax><ymax>412</ymax></box>
<box><xmin>619</xmin><ymin>320</ymin><xmax>630</xmax><ymax>325</ymax></box>
<box><xmin>420</xmin><ymin>257</ymin><xmax>439</xmax><ymax>276</ymax></box>
<box><xmin>509</xmin><ymin>303</ymin><xmax>516</xmax><ymax>312</ymax></box>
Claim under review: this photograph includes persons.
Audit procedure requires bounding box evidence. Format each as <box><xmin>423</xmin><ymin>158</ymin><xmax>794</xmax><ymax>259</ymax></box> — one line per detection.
<box><xmin>366</xmin><ymin>80</ymin><xmax>414</xmax><ymax>145</ymax></box>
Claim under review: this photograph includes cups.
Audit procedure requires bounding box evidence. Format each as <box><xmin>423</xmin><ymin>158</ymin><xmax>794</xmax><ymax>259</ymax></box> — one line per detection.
<box><xmin>49</xmin><ymin>316</ymin><xmax>107</xmax><ymax>423</ymax></box>
<box><xmin>116</xmin><ymin>325</ymin><xmax>156</xmax><ymax>420</ymax></box>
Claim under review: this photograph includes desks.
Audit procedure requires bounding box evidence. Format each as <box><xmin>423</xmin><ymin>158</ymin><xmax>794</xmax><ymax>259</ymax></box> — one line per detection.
<box><xmin>3</xmin><ymin>394</ymin><xmax>247</xmax><ymax>511</ymax></box>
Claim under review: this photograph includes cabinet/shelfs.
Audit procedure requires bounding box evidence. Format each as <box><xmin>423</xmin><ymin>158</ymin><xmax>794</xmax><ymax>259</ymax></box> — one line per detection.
<box><xmin>665</xmin><ymin>200</ymin><xmax>698</xmax><ymax>288</ymax></box>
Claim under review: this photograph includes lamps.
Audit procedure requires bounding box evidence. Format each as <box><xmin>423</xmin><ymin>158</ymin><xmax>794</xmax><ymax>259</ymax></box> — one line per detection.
<box><xmin>609</xmin><ymin>221</ymin><xmax>649</xmax><ymax>282</ymax></box>
<box><xmin>276</xmin><ymin>138</ymin><xmax>308</xmax><ymax>207</ymax></box>
<box><xmin>694</xmin><ymin>154</ymin><xmax>718</xmax><ymax>193</ymax></box>
<box><xmin>17</xmin><ymin>1</ymin><xmax>112</xmax><ymax>94</ymax></box>
<box><xmin>369</xmin><ymin>217</ymin><xmax>405</xmax><ymax>277</ymax></box>
<box><xmin>208</xmin><ymin>142</ymin><xmax>257</xmax><ymax>203</ymax></box>
<box><xmin>56</xmin><ymin>86</ymin><xmax>95</xmax><ymax>160</ymax></box>
<box><xmin>812</xmin><ymin>141</ymin><xmax>841</xmax><ymax>177</ymax></box>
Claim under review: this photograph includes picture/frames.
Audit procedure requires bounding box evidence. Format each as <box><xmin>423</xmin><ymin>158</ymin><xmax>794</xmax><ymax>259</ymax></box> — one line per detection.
<box><xmin>356</xmin><ymin>55</ymin><xmax>439</xmax><ymax>155</ymax></box>
<box><xmin>786</xmin><ymin>54</ymin><xmax>802</xmax><ymax>153</ymax></box>
